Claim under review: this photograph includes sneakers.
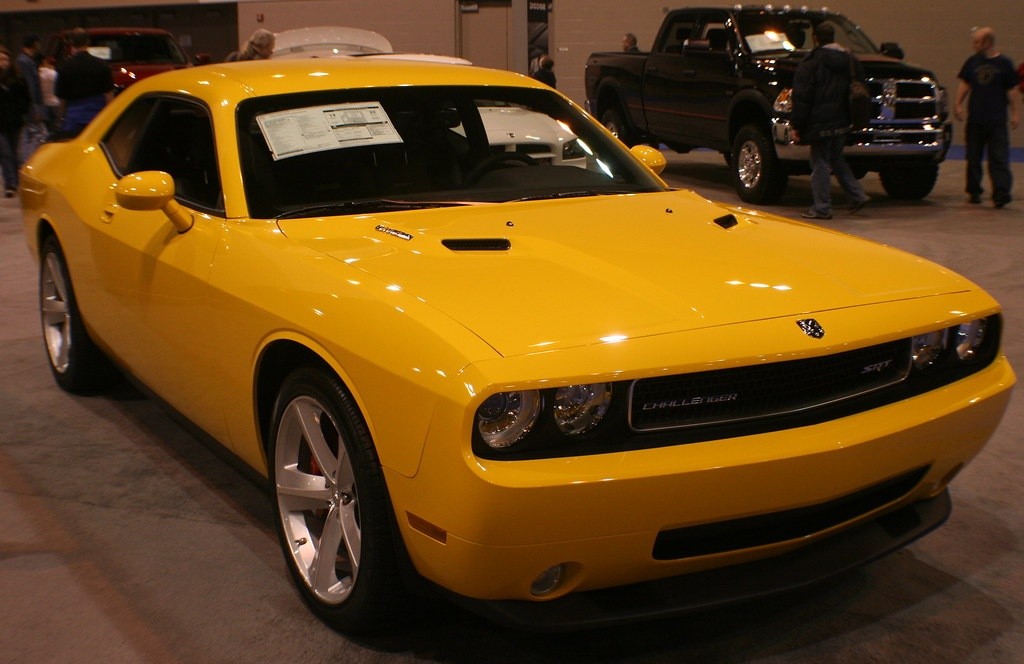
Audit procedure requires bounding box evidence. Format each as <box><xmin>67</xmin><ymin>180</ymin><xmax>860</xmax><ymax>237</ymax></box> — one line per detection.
<box><xmin>848</xmin><ymin>193</ymin><xmax>869</xmax><ymax>214</ymax></box>
<box><xmin>800</xmin><ymin>207</ymin><xmax>832</xmax><ymax>219</ymax></box>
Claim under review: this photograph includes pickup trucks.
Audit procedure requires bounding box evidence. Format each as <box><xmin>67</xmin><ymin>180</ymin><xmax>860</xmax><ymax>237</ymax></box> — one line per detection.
<box><xmin>584</xmin><ymin>5</ymin><xmax>955</xmax><ymax>206</ymax></box>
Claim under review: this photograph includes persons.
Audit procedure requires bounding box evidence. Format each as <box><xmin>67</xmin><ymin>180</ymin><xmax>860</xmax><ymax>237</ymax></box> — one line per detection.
<box><xmin>789</xmin><ymin>23</ymin><xmax>871</xmax><ymax>219</ymax></box>
<box><xmin>0</xmin><ymin>33</ymin><xmax>74</xmax><ymax>197</ymax></box>
<box><xmin>955</xmin><ymin>25</ymin><xmax>1024</xmax><ymax>209</ymax></box>
<box><xmin>236</xmin><ymin>29</ymin><xmax>274</xmax><ymax>61</ymax></box>
<box><xmin>621</xmin><ymin>32</ymin><xmax>643</xmax><ymax>53</ymax></box>
<box><xmin>534</xmin><ymin>56</ymin><xmax>557</xmax><ymax>88</ymax></box>
<box><xmin>53</xmin><ymin>27</ymin><xmax>115</xmax><ymax>140</ymax></box>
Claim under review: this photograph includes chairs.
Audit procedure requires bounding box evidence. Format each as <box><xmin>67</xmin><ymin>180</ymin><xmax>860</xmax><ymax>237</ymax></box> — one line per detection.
<box><xmin>367</xmin><ymin>110</ymin><xmax>438</xmax><ymax>196</ymax></box>
<box><xmin>706</xmin><ymin>27</ymin><xmax>730</xmax><ymax>51</ymax></box>
<box><xmin>666</xmin><ymin>27</ymin><xmax>692</xmax><ymax>53</ymax></box>
<box><xmin>788</xmin><ymin>32</ymin><xmax>806</xmax><ymax>49</ymax></box>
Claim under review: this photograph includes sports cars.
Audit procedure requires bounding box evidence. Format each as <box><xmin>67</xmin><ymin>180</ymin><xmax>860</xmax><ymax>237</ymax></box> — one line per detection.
<box><xmin>40</xmin><ymin>30</ymin><xmax>1013</xmax><ymax>638</ymax></box>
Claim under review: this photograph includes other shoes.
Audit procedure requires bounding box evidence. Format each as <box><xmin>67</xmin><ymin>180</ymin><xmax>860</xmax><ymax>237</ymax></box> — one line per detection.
<box><xmin>970</xmin><ymin>195</ymin><xmax>981</xmax><ymax>203</ymax></box>
<box><xmin>995</xmin><ymin>195</ymin><xmax>1012</xmax><ymax>208</ymax></box>
<box><xmin>5</xmin><ymin>189</ymin><xmax>15</xmax><ymax>198</ymax></box>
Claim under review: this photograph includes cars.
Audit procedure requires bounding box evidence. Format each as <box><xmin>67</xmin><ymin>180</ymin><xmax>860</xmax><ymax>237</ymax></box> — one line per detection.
<box><xmin>236</xmin><ymin>23</ymin><xmax>591</xmax><ymax>173</ymax></box>
<box><xmin>47</xmin><ymin>26</ymin><xmax>212</xmax><ymax>100</ymax></box>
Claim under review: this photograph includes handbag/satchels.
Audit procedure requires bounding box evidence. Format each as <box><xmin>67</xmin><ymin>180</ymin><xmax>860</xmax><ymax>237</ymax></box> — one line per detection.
<box><xmin>849</xmin><ymin>94</ymin><xmax>880</xmax><ymax>128</ymax></box>
